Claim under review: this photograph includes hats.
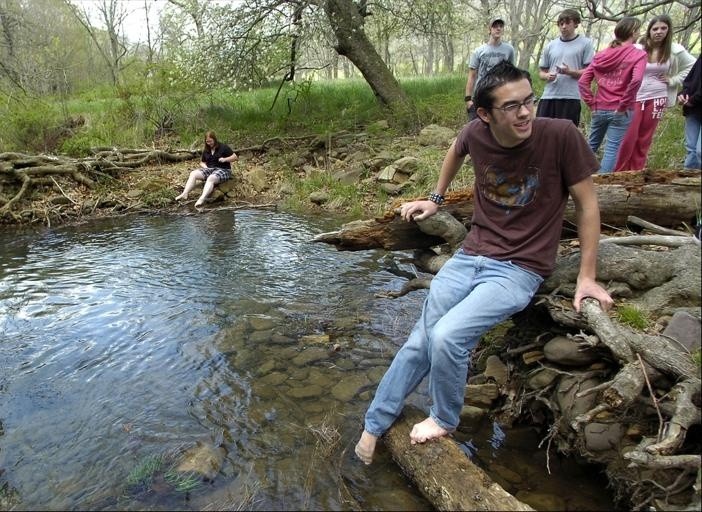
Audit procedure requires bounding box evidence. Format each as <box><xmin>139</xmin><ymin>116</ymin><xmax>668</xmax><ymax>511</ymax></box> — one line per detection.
<box><xmin>488</xmin><ymin>17</ymin><xmax>505</xmax><ymax>28</ymax></box>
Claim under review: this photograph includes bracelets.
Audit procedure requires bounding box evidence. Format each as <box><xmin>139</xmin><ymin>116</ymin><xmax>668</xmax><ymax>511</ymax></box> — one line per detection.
<box><xmin>428</xmin><ymin>193</ymin><xmax>444</xmax><ymax>206</ymax></box>
<box><xmin>465</xmin><ymin>96</ymin><xmax>472</xmax><ymax>102</ymax></box>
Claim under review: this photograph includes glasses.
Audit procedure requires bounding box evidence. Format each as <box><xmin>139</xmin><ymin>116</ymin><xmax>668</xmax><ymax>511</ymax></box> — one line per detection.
<box><xmin>485</xmin><ymin>95</ymin><xmax>537</xmax><ymax>114</ymax></box>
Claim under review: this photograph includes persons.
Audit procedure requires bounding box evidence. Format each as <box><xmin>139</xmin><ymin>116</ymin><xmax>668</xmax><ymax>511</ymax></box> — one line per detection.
<box><xmin>175</xmin><ymin>131</ymin><xmax>237</xmax><ymax>207</ymax></box>
<box><xmin>677</xmin><ymin>51</ymin><xmax>702</xmax><ymax>169</ymax></box>
<box><xmin>578</xmin><ymin>17</ymin><xmax>649</xmax><ymax>172</ymax></box>
<box><xmin>353</xmin><ymin>60</ymin><xmax>616</xmax><ymax>465</ymax></box>
<box><xmin>613</xmin><ymin>14</ymin><xmax>697</xmax><ymax>171</ymax></box>
<box><xmin>465</xmin><ymin>17</ymin><xmax>515</xmax><ymax>122</ymax></box>
<box><xmin>536</xmin><ymin>9</ymin><xmax>594</xmax><ymax>129</ymax></box>
